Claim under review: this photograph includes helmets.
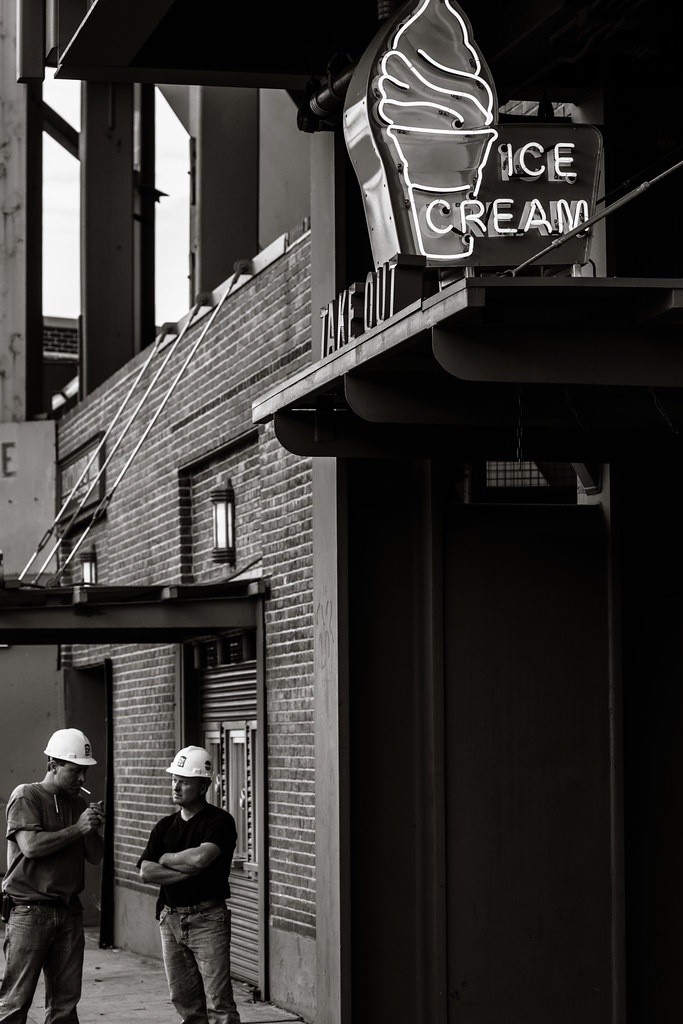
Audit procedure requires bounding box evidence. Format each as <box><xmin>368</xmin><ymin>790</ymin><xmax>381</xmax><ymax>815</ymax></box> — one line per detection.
<box><xmin>165</xmin><ymin>745</ymin><xmax>214</xmax><ymax>784</ymax></box>
<box><xmin>43</xmin><ymin>727</ymin><xmax>97</xmax><ymax>766</ymax></box>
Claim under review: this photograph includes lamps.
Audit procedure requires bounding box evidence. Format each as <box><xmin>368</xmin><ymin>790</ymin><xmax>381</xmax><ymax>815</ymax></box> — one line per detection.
<box><xmin>80</xmin><ymin>543</ymin><xmax>97</xmax><ymax>587</ymax></box>
<box><xmin>210</xmin><ymin>477</ymin><xmax>236</xmax><ymax>568</ymax></box>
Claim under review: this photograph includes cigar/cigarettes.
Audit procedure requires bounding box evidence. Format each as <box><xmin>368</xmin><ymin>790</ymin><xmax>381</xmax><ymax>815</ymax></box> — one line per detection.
<box><xmin>79</xmin><ymin>786</ymin><xmax>92</xmax><ymax>795</ymax></box>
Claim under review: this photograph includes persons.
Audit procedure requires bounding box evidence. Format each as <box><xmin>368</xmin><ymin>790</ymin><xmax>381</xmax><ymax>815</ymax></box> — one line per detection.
<box><xmin>133</xmin><ymin>745</ymin><xmax>241</xmax><ymax>1024</ymax></box>
<box><xmin>1</xmin><ymin>728</ymin><xmax>109</xmax><ymax>1024</ymax></box>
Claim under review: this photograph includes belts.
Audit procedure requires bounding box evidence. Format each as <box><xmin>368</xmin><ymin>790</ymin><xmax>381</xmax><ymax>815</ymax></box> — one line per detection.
<box><xmin>14</xmin><ymin>898</ymin><xmax>75</xmax><ymax>909</ymax></box>
<box><xmin>163</xmin><ymin>901</ymin><xmax>210</xmax><ymax>914</ymax></box>
